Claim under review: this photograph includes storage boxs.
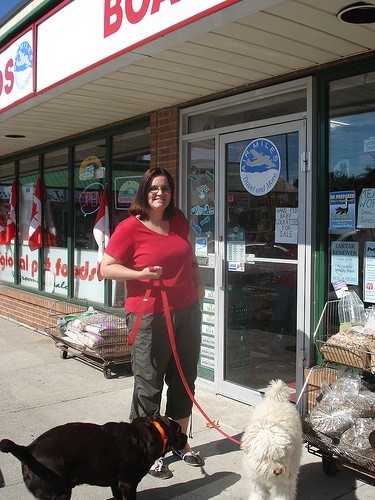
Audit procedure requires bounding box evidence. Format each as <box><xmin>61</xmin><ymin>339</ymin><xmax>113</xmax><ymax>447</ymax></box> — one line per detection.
<box><xmin>200</xmin><ymin>284</ymin><xmax>253</xmax><ymax>379</ymax></box>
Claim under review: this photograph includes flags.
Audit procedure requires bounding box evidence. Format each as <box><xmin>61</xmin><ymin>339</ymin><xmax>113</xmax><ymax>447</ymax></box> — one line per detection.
<box><xmin>28</xmin><ymin>173</ymin><xmax>56</xmax><ymax>251</ymax></box>
<box><xmin>92</xmin><ymin>182</ymin><xmax>115</xmax><ymax>283</ymax></box>
<box><xmin>0</xmin><ymin>176</ymin><xmax>17</xmax><ymax>246</ymax></box>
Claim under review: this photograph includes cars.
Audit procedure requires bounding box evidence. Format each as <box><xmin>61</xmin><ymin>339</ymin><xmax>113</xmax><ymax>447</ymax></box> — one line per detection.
<box><xmin>230</xmin><ymin>244</ymin><xmax>296</xmax><ymax>277</ymax></box>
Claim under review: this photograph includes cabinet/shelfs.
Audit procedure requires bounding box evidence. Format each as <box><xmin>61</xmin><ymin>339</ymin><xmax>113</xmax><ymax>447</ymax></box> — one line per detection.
<box><xmin>228</xmin><ymin>202</ymin><xmax>272</xmax><ymax>255</ymax></box>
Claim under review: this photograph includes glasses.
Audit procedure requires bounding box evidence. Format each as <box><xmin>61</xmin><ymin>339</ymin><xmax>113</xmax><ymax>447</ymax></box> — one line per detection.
<box><xmin>148</xmin><ymin>186</ymin><xmax>171</xmax><ymax>194</ymax></box>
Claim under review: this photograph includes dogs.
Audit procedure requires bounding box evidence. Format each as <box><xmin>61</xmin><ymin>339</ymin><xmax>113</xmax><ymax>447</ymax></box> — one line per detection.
<box><xmin>0</xmin><ymin>416</ymin><xmax>188</xmax><ymax>500</ymax></box>
<box><xmin>240</xmin><ymin>379</ymin><xmax>303</xmax><ymax>500</ymax></box>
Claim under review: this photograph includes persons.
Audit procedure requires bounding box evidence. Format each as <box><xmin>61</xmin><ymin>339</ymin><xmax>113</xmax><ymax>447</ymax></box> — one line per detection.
<box><xmin>99</xmin><ymin>167</ymin><xmax>205</xmax><ymax>480</ymax></box>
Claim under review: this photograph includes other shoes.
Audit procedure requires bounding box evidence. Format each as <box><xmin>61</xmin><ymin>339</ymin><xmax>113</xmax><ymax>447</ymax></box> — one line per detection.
<box><xmin>171</xmin><ymin>446</ymin><xmax>204</xmax><ymax>466</ymax></box>
<box><xmin>147</xmin><ymin>457</ymin><xmax>173</xmax><ymax>479</ymax></box>
